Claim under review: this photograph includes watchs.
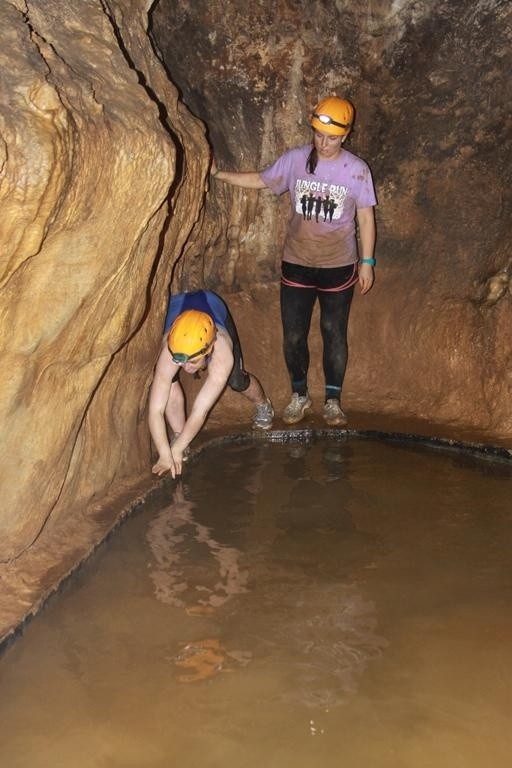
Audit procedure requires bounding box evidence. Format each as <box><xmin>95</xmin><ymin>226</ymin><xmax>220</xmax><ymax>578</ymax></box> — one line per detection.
<box><xmin>359</xmin><ymin>258</ymin><xmax>375</xmax><ymax>267</ymax></box>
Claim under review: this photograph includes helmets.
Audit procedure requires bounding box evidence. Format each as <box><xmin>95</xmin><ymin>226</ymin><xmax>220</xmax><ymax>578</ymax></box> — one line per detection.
<box><xmin>310</xmin><ymin>96</ymin><xmax>354</xmax><ymax>136</ymax></box>
<box><xmin>168</xmin><ymin>310</ymin><xmax>217</xmax><ymax>361</ymax></box>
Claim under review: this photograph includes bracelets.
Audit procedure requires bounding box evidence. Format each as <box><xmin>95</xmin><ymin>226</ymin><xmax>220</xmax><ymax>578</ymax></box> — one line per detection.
<box><xmin>212</xmin><ymin>169</ymin><xmax>220</xmax><ymax>178</ymax></box>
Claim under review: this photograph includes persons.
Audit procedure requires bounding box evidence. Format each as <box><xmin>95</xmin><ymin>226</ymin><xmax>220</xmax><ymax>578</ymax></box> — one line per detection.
<box><xmin>146</xmin><ymin>287</ymin><xmax>276</xmax><ymax>480</ymax></box>
<box><xmin>209</xmin><ymin>95</ymin><xmax>378</xmax><ymax>429</ymax></box>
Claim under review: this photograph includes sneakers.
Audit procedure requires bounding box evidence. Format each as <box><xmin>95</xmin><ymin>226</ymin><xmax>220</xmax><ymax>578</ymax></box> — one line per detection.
<box><xmin>283</xmin><ymin>389</ymin><xmax>312</xmax><ymax>425</ymax></box>
<box><xmin>322</xmin><ymin>398</ymin><xmax>348</xmax><ymax>427</ymax></box>
<box><xmin>170</xmin><ymin>432</ymin><xmax>190</xmax><ymax>461</ymax></box>
<box><xmin>251</xmin><ymin>396</ymin><xmax>274</xmax><ymax>431</ymax></box>
